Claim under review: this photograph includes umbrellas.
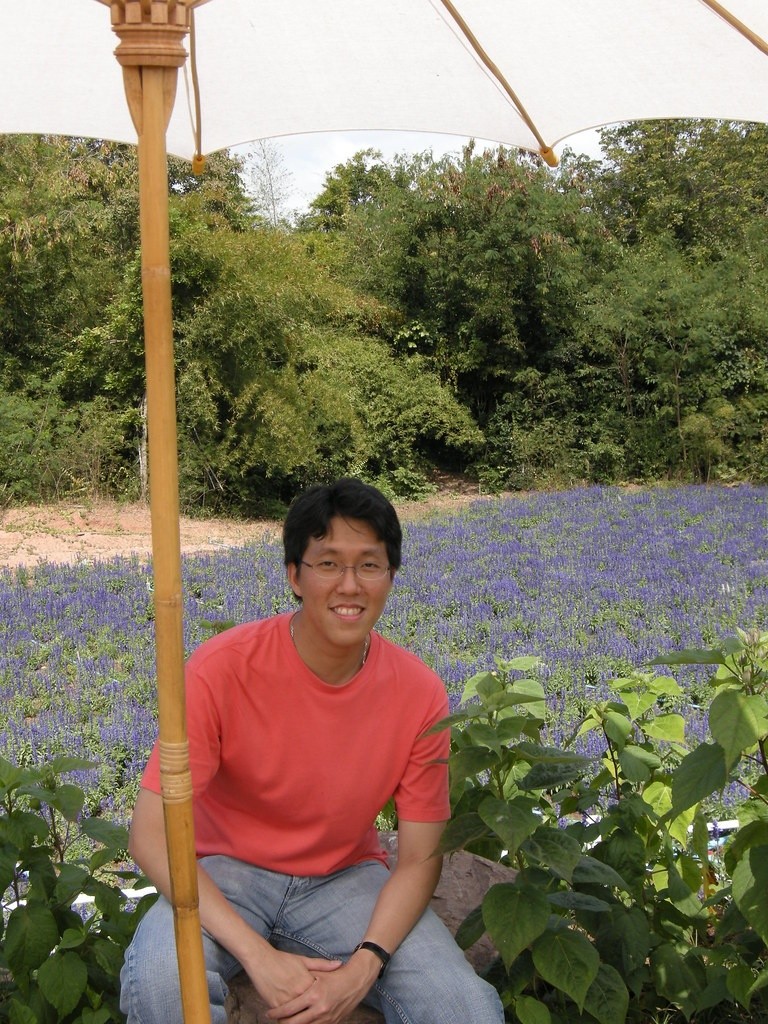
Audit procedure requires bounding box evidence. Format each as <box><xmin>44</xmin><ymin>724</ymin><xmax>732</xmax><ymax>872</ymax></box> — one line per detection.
<box><xmin>0</xmin><ymin>6</ymin><xmax>768</xmax><ymax>1021</ymax></box>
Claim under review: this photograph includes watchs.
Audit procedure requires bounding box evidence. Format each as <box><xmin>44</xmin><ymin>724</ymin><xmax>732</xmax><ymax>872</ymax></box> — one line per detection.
<box><xmin>355</xmin><ymin>941</ymin><xmax>393</xmax><ymax>980</ymax></box>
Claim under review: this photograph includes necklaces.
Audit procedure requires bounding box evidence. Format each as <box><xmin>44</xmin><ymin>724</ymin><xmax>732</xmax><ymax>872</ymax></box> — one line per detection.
<box><xmin>289</xmin><ymin>622</ymin><xmax>368</xmax><ymax>668</ymax></box>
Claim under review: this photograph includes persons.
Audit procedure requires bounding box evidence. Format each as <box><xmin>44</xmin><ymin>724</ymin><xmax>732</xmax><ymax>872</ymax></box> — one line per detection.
<box><xmin>119</xmin><ymin>477</ymin><xmax>506</xmax><ymax>1024</ymax></box>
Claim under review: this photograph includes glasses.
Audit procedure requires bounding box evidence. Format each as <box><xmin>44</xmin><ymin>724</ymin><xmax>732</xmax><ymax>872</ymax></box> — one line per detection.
<box><xmin>300</xmin><ymin>560</ymin><xmax>392</xmax><ymax>580</ymax></box>
